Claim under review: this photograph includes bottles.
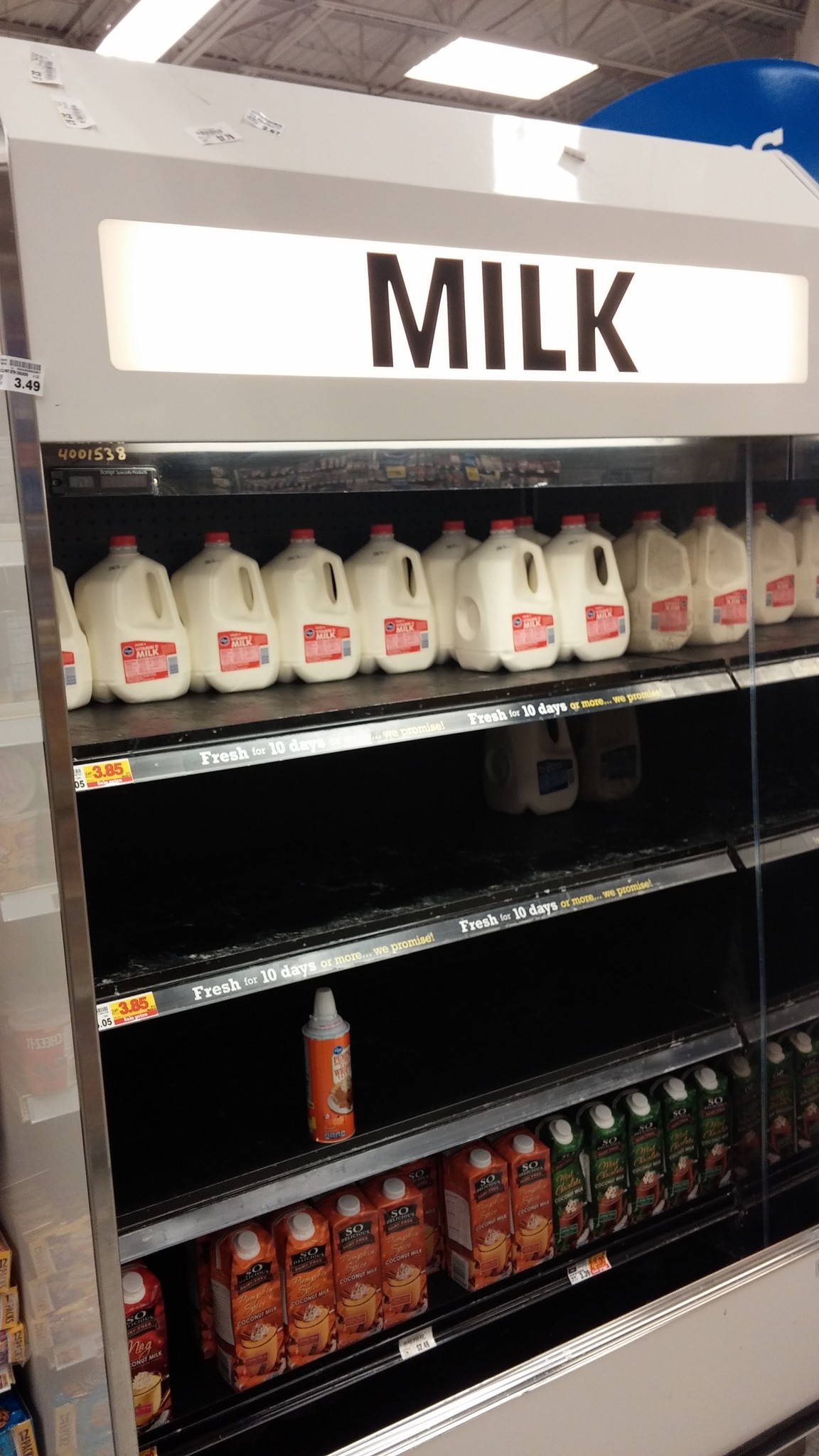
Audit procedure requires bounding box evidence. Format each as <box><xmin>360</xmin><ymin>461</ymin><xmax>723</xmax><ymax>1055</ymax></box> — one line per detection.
<box><xmin>455</xmin><ymin>519</ymin><xmax>559</xmax><ymax>674</ymax></box>
<box><xmin>783</xmin><ymin>497</ymin><xmax>818</xmax><ymax>617</ymax></box>
<box><xmin>170</xmin><ymin>531</ymin><xmax>277</xmax><ymax>695</ymax></box>
<box><xmin>482</xmin><ymin>706</ymin><xmax>645</xmax><ymax>819</ymax></box>
<box><xmin>516</xmin><ymin>512</ymin><xmax>631</xmax><ymax>663</ymax></box>
<box><xmin>345</xmin><ymin>526</ymin><xmax>437</xmax><ymax>674</ymax></box>
<box><xmin>676</xmin><ymin>508</ymin><xmax>754</xmax><ymax>646</ymax></box>
<box><xmin>731</xmin><ymin>502</ymin><xmax>800</xmax><ymax>625</ymax></box>
<box><xmin>410</xmin><ymin>520</ymin><xmax>483</xmax><ymax>665</ymax></box>
<box><xmin>260</xmin><ymin>528</ymin><xmax>361</xmax><ymax>683</ymax></box>
<box><xmin>302</xmin><ymin>986</ymin><xmax>356</xmax><ymax>1145</ymax></box>
<box><xmin>51</xmin><ymin>565</ymin><xmax>92</xmax><ymax>710</ymax></box>
<box><xmin>601</xmin><ymin>510</ymin><xmax>695</xmax><ymax>654</ymax></box>
<box><xmin>73</xmin><ymin>537</ymin><xmax>190</xmax><ymax>704</ymax></box>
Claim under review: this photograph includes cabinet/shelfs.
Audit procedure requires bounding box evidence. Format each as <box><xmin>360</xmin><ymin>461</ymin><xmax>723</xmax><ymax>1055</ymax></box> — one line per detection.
<box><xmin>0</xmin><ymin>164</ymin><xmax>819</xmax><ymax>1456</ymax></box>
